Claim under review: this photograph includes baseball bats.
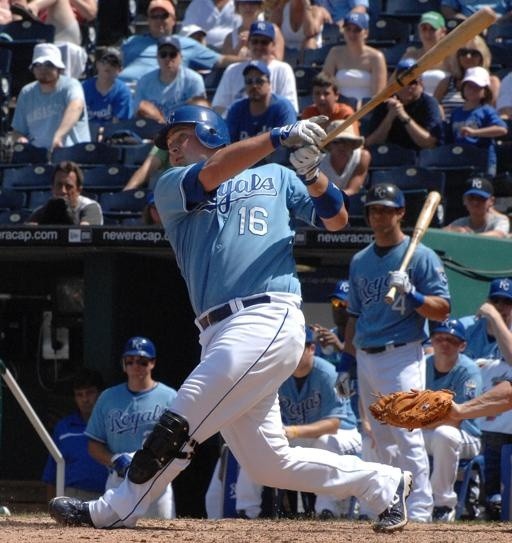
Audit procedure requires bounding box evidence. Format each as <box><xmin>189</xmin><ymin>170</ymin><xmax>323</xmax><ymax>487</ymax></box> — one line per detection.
<box><xmin>384</xmin><ymin>191</ymin><xmax>441</xmax><ymax>303</ymax></box>
<box><xmin>316</xmin><ymin>6</ymin><xmax>496</xmax><ymax>151</ymax></box>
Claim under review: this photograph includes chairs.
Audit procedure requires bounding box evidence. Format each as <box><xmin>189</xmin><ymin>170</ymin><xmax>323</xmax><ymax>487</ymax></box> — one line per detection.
<box><xmin>426</xmin><ymin>431</ymin><xmax>511</xmax><ymax>523</ymax></box>
<box><xmin>0</xmin><ymin>0</ymin><xmax>512</xmax><ymax>243</ymax></box>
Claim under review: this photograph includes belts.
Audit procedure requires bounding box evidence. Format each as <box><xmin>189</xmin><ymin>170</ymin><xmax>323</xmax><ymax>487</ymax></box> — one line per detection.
<box><xmin>361</xmin><ymin>339</ymin><xmax>407</xmax><ymax>355</ymax></box>
<box><xmin>196</xmin><ymin>293</ymin><xmax>272</xmax><ymax>333</ymax></box>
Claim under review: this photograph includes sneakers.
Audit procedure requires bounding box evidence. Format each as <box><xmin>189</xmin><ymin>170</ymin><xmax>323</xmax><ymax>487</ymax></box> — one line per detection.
<box><xmin>49</xmin><ymin>495</ymin><xmax>95</xmax><ymax>529</ymax></box>
<box><xmin>370</xmin><ymin>467</ymin><xmax>411</xmax><ymax>534</ymax></box>
<box><xmin>462</xmin><ymin>480</ymin><xmax>503</xmax><ymax>519</ymax></box>
<box><xmin>431</xmin><ymin>505</ymin><xmax>451</xmax><ymax>526</ymax></box>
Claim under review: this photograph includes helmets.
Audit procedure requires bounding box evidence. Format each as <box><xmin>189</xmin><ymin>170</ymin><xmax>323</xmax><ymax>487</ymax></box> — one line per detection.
<box><xmin>121</xmin><ymin>336</ymin><xmax>156</xmax><ymax>369</ymax></box>
<box><xmin>154</xmin><ymin>106</ymin><xmax>230</xmax><ymax>149</ymax></box>
<box><xmin>363</xmin><ymin>182</ymin><xmax>406</xmax><ymax>211</ymax></box>
<box><xmin>427</xmin><ymin>318</ymin><xmax>467</xmax><ymax>340</ymax></box>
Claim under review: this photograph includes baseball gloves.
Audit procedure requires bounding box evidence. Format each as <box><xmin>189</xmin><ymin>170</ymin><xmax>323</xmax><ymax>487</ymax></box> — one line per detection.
<box><xmin>369</xmin><ymin>387</ymin><xmax>456</xmax><ymax>433</ymax></box>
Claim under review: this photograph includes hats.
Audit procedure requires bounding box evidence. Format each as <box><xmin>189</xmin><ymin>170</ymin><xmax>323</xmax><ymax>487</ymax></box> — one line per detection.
<box><xmin>243</xmin><ymin>18</ymin><xmax>277</xmax><ymax>79</ymax></box>
<box><xmin>328</xmin><ymin>279</ymin><xmax>350</xmax><ymax>300</ymax></box>
<box><xmin>304</xmin><ymin>324</ymin><xmax>316</xmax><ymax>344</ymax></box>
<box><xmin>488</xmin><ymin>279</ymin><xmax>512</xmax><ymax>298</ymax></box>
<box><xmin>28</xmin><ymin>42</ymin><xmax>66</xmax><ymax>72</ymax></box>
<box><xmin>342</xmin><ymin>11</ymin><xmax>495</xmax><ymax>89</ymax></box>
<box><xmin>100</xmin><ymin>46</ymin><xmax>122</xmax><ymax>63</ymax></box>
<box><xmin>321</xmin><ymin>118</ymin><xmax>365</xmax><ymax>155</ymax></box>
<box><xmin>462</xmin><ymin>178</ymin><xmax>496</xmax><ymax>198</ymax></box>
<box><xmin>148</xmin><ymin>0</ymin><xmax>207</xmax><ymax>51</ymax></box>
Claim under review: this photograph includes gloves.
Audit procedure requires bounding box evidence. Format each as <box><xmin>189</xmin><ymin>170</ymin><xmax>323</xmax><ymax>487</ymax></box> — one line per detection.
<box><xmin>288</xmin><ymin>145</ymin><xmax>322</xmax><ymax>186</ymax></box>
<box><xmin>386</xmin><ymin>268</ymin><xmax>417</xmax><ymax>300</ymax></box>
<box><xmin>111</xmin><ymin>450</ymin><xmax>137</xmax><ymax>478</ymax></box>
<box><xmin>334</xmin><ymin>353</ymin><xmax>355</xmax><ymax>400</ymax></box>
<box><xmin>270</xmin><ymin>114</ymin><xmax>331</xmax><ymax>150</ymax></box>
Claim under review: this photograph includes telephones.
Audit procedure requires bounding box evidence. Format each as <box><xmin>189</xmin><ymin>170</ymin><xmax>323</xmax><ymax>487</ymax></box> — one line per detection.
<box><xmin>41</xmin><ymin>310</ymin><xmax>71</xmax><ymax>360</ymax></box>
